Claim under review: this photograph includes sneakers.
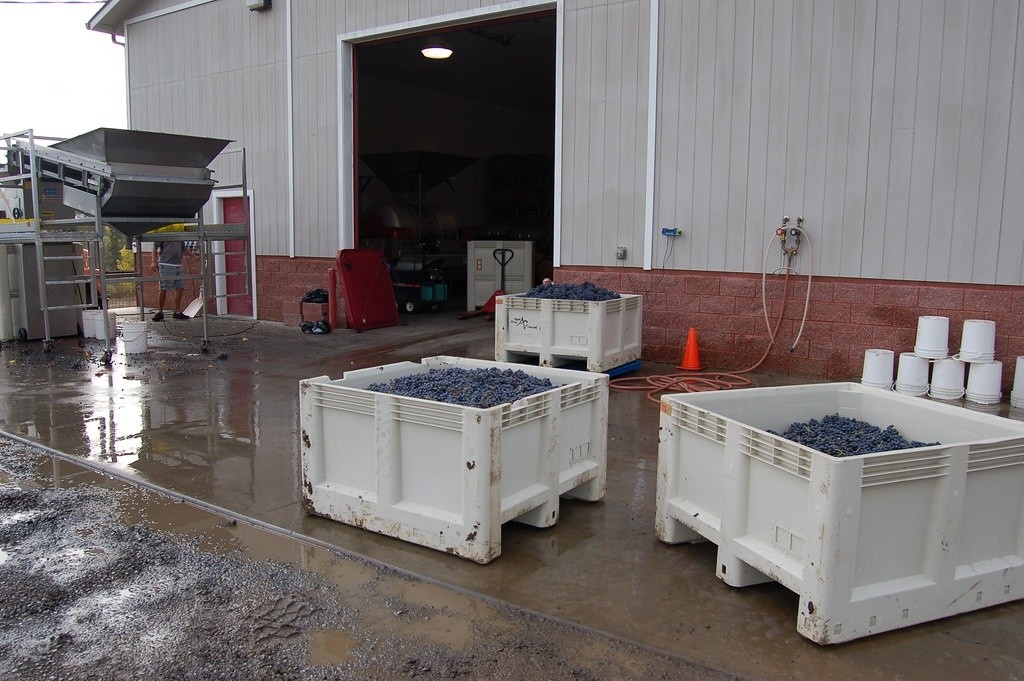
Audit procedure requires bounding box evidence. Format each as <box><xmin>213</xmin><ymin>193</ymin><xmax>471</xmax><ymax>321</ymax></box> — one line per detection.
<box><xmin>173</xmin><ymin>313</ymin><xmax>189</xmax><ymax>319</ymax></box>
<box><xmin>152</xmin><ymin>312</ymin><xmax>164</xmax><ymax>322</ymax></box>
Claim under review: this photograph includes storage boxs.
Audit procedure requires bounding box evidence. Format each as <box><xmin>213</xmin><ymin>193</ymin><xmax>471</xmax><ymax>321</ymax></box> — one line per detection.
<box><xmin>283</xmin><ymin>313</ymin><xmax>303</xmax><ymax>325</ymax></box>
<box><xmin>304</xmin><ymin>315</ymin><xmax>328</xmax><ymax>322</ymax></box>
<box><xmin>299</xmin><ymin>355</ymin><xmax>609</xmax><ymax>563</ymax></box>
<box><xmin>282</xmin><ymin>301</ymin><xmax>303</xmax><ymax>314</ymax></box>
<box><xmin>302</xmin><ymin>302</ymin><xmax>329</xmax><ymax>316</ymax></box>
<box><xmin>656</xmin><ymin>382</ymin><xmax>1024</xmax><ymax>644</ymax></box>
<box><xmin>495</xmin><ymin>292</ymin><xmax>643</xmax><ymax>372</ymax></box>
<box><xmin>467</xmin><ymin>241</ymin><xmax>533</xmax><ymax>312</ymax></box>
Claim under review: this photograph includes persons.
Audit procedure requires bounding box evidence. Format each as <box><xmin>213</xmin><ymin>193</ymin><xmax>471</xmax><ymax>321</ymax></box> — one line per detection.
<box><xmin>150</xmin><ymin>240</ymin><xmax>202</xmax><ymax>319</ymax></box>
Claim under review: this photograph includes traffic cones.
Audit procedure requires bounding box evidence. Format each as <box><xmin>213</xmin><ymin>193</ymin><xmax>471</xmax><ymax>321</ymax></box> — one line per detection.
<box><xmin>675</xmin><ymin>327</ymin><xmax>702</xmax><ymax>372</ymax></box>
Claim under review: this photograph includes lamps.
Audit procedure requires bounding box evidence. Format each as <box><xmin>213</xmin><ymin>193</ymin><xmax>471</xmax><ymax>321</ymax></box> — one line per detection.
<box><xmin>421</xmin><ymin>34</ymin><xmax>453</xmax><ymax>59</ymax></box>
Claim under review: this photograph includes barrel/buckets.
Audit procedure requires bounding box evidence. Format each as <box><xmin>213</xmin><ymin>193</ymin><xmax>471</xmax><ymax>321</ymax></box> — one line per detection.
<box><xmin>82</xmin><ymin>310</ymin><xmax>103</xmax><ymax>338</ymax></box>
<box><xmin>964</xmin><ymin>359</ymin><xmax>1003</xmax><ymax>405</ymax></box>
<box><xmin>119</xmin><ymin>321</ymin><xmax>148</xmax><ymax>354</ymax></box>
<box><xmin>891</xmin><ymin>352</ymin><xmax>930</xmax><ymax>396</ymax></box>
<box><xmin>952</xmin><ymin>319</ymin><xmax>996</xmax><ymax>364</ymax></box>
<box><xmin>913</xmin><ymin>315</ymin><xmax>949</xmax><ymax>363</ymax></box>
<box><xmin>860</xmin><ymin>349</ymin><xmax>895</xmax><ymax>391</ymax></box>
<box><xmin>1009</xmin><ymin>356</ymin><xmax>1024</xmax><ymax>408</ymax></box>
<box><xmin>93</xmin><ymin>311</ymin><xmax>117</xmax><ymax>340</ymax></box>
<box><xmin>926</xmin><ymin>356</ymin><xmax>966</xmax><ymax>401</ymax></box>
